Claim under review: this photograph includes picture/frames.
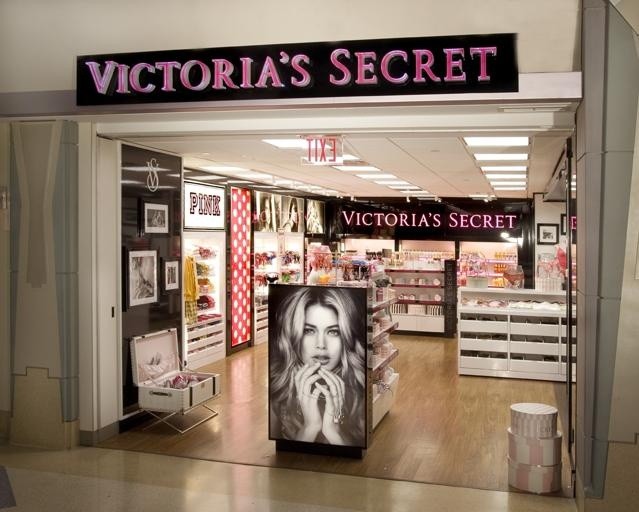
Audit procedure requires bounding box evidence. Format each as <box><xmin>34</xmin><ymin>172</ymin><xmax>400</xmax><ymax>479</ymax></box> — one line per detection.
<box><xmin>124</xmin><ymin>197</ymin><xmax>181</xmax><ymax>313</ymax></box>
<box><xmin>537</xmin><ymin>223</ymin><xmax>559</xmax><ymax>245</ymax></box>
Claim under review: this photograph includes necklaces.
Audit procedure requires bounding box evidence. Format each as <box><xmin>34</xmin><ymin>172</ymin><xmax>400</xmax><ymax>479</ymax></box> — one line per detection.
<box><xmin>301</xmin><ymin>391</ymin><xmax>347</xmax><ymax>425</ymax></box>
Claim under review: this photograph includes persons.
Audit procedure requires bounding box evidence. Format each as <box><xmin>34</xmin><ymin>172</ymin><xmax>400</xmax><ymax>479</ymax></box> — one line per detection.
<box><xmin>282</xmin><ymin>197</ymin><xmax>299</xmax><ymax>232</ymax></box>
<box><xmin>268</xmin><ymin>287</ymin><xmax>366</xmax><ymax>448</ymax></box>
<box><xmin>257</xmin><ymin>196</ymin><xmax>273</xmax><ymax>232</ymax></box>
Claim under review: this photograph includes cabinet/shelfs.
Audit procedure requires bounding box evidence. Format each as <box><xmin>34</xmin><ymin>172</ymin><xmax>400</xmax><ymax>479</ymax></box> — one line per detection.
<box><xmin>182</xmin><ymin>231</ymin><xmax>225</xmax><ymax>371</ymax></box>
<box><xmin>254</xmin><ymin>305</ymin><xmax>269</xmax><ymax>345</ymax></box>
<box><xmin>487</xmin><ymin>261</ymin><xmax>517</xmax><ymax>288</ymax></box>
<box><xmin>385</xmin><ymin>268</ymin><xmax>444</xmax><ymax>333</ymax></box>
<box><xmin>256</xmin><ymin>232</ymin><xmax>304</xmax><ymax>308</ymax></box>
<box><xmin>367</xmin><ymin>296</ymin><xmax>403</xmax><ymax>434</ymax></box>
<box><xmin>458</xmin><ymin>289</ymin><xmax>577</xmax><ymax>383</ymax></box>
